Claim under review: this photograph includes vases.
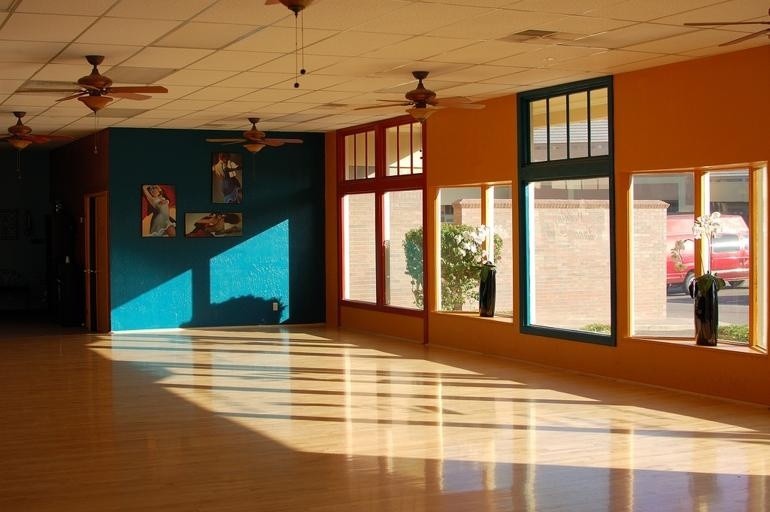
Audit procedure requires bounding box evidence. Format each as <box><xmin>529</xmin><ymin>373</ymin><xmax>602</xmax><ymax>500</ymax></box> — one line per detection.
<box><xmin>479</xmin><ymin>264</ymin><xmax>496</xmax><ymax>317</ymax></box>
<box><xmin>689</xmin><ymin>276</ymin><xmax>719</xmax><ymax>346</ymax></box>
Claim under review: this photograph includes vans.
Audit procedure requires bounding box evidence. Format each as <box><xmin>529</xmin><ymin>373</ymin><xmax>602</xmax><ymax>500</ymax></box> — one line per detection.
<box><xmin>666</xmin><ymin>212</ymin><xmax>750</xmax><ymax>295</ymax></box>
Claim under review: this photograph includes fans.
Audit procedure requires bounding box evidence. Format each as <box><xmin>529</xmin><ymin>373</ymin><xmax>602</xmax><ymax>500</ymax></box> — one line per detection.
<box><xmin>16</xmin><ymin>54</ymin><xmax>168</xmax><ymax>102</ymax></box>
<box><xmin>206</xmin><ymin>117</ymin><xmax>304</xmax><ymax>147</ymax></box>
<box><xmin>353</xmin><ymin>70</ymin><xmax>486</xmax><ymax>111</ymax></box>
<box><xmin>0</xmin><ymin>111</ymin><xmax>75</xmax><ymax>143</ymax></box>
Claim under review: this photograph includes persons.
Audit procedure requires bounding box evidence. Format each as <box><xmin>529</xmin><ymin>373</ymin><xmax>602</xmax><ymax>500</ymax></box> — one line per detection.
<box><xmin>190</xmin><ymin>213</ymin><xmax>239</xmax><ymax>235</ymax></box>
<box><xmin>142</xmin><ymin>184</ymin><xmax>175</xmax><ymax>237</ymax></box>
<box><xmin>213</xmin><ymin>152</ymin><xmax>243</xmax><ymax>204</ymax></box>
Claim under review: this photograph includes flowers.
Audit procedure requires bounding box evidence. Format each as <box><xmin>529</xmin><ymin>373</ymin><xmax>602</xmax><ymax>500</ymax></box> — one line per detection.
<box><xmin>668</xmin><ymin>210</ymin><xmax>726</xmax><ymax>294</ymax></box>
<box><xmin>455</xmin><ymin>224</ymin><xmax>492</xmax><ymax>264</ymax></box>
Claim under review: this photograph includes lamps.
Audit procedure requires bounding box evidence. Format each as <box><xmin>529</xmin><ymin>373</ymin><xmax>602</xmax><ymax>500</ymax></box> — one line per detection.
<box><xmin>6</xmin><ymin>140</ymin><xmax>33</xmax><ymax>179</ymax></box>
<box><xmin>405</xmin><ymin>106</ymin><xmax>437</xmax><ymax>124</ymax></box>
<box><xmin>77</xmin><ymin>96</ymin><xmax>114</xmax><ymax>155</ymax></box>
<box><xmin>243</xmin><ymin>143</ymin><xmax>265</xmax><ymax>155</ymax></box>
<box><xmin>279</xmin><ymin>1</ymin><xmax>313</xmax><ymax>88</ymax></box>
